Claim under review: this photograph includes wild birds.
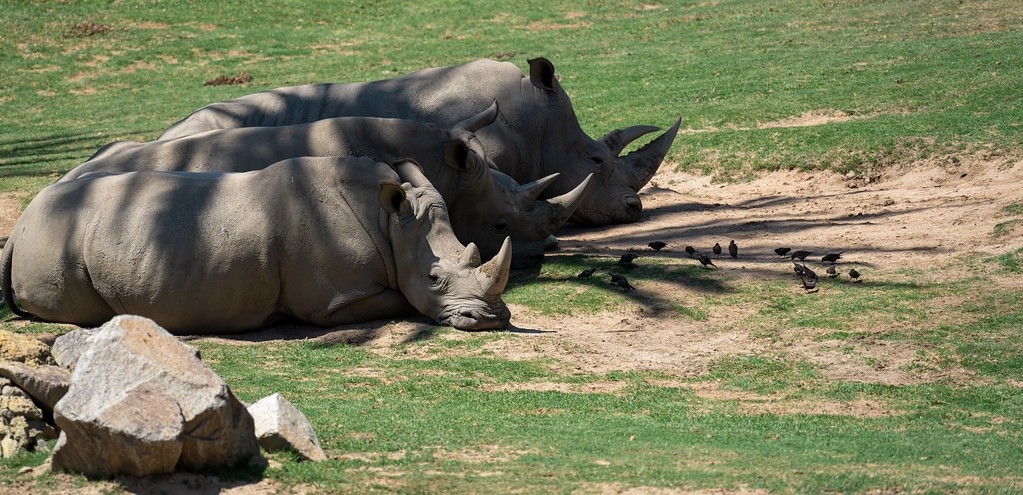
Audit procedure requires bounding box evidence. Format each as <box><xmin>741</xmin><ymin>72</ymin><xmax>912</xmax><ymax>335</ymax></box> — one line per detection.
<box><xmin>648</xmin><ymin>241</ymin><xmax>668</xmax><ymax>251</ymax></box>
<box><xmin>848</xmin><ymin>269</ymin><xmax>861</xmax><ymax>281</ymax></box>
<box><xmin>686</xmin><ymin>246</ymin><xmax>699</xmax><ymax>256</ymax></box>
<box><xmin>696</xmin><ymin>254</ymin><xmax>717</xmax><ymax>269</ymax></box>
<box><xmin>577</xmin><ymin>268</ymin><xmax>597</xmax><ymax>278</ymax></box>
<box><xmin>826</xmin><ymin>266</ymin><xmax>837</xmax><ymax>274</ymax></box>
<box><xmin>822</xmin><ymin>250</ymin><xmax>845</xmax><ymax>264</ymax></box>
<box><xmin>792</xmin><ymin>260</ymin><xmax>819</xmax><ymax>291</ymax></box>
<box><xmin>606</xmin><ymin>272</ymin><xmax>635</xmax><ymax>292</ymax></box>
<box><xmin>613</xmin><ymin>253</ymin><xmax>640</xmax><ymax>270</ymax></box>
<box><xmin>728</xmin><ymin>240</ymin><xmax>738</xmax><ymax>259</ymax></box>
<box><xmin>713</xmin><ymin>243</ymin><xmax>722</xmax><ymax>258</ymax></box>
<box><xmin>773</xmin><ymin>247</ymin><xmax>792</xmax><ymax>258</ymax></box>
<box><xmin>791</xmin><ymin>251</ymin><xmax>813</xmax><ymax>261</ymax></box>
